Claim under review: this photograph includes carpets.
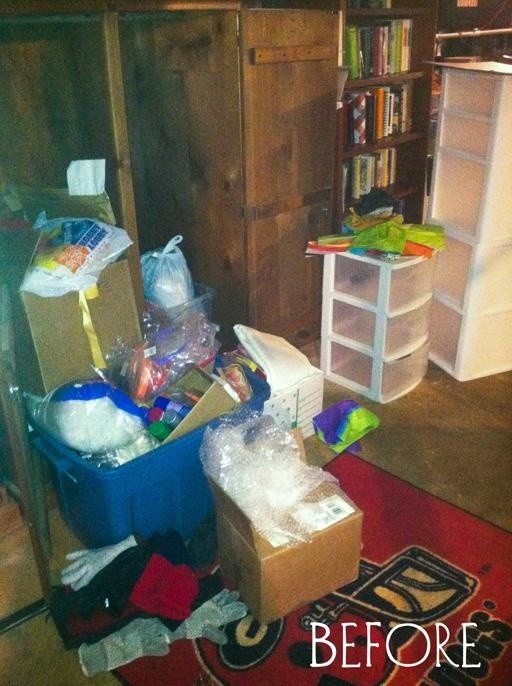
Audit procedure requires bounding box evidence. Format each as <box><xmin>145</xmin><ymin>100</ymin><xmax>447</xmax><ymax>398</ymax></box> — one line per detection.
<box><xmin>77</xmin><ymin>449</ymin><xmax>512</xmax><ymax>686</ymax></box>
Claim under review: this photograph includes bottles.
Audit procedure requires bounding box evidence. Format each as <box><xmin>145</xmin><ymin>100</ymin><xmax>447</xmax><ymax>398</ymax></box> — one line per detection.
<box><xmin>149</xmin><ymin>396</ymin><xmax>190</xmax><ymax>441</ymax></box>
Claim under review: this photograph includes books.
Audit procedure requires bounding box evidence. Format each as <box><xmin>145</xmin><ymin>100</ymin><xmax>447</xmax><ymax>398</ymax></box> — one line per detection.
<box><xmin>342</xmin><ymin>0</ymin><xmax>414</xmax><ymax>200</ymax></box>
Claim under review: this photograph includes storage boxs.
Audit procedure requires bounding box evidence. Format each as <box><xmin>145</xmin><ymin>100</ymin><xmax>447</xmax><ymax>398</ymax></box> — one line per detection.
<box><xmin>189</xmin><ymin>422</ymin><xmax>362</xmax><ymax>623</ymax></box>
<box><xmin>25</xmin><ymin>355</ymin><xmax>269</xmax><ymax>553</ymax></box>
<box><xmin>0</xmin><ymin>224</ymin><xmax>142</xmax><ymax>395</ymax></box>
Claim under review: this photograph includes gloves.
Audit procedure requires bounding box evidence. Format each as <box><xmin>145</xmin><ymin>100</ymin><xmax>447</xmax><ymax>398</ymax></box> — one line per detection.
<box><xmin>60</xmin><ymin>533</ymin><xmax>139</xmax><ymax>594</ymax></box>
<box><xmin>79</xmin><ymin>616</ymin><xmax>174</xmax><ymax>677</ymax></box>
<box><xmin>170</xmin><ymin>587</ymin><xmax>250</xmax><ymax>646</ymax></box>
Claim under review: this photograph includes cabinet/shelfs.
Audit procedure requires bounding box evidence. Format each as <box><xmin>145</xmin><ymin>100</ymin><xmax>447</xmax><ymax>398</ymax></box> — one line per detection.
<box><xmin>239</xmin><ymin>3</ymin><xmax>343</xmax><ymax>335</ymax></box>
<box><xmin>0</xmin><ymin>3</ymin><xmax>248</xmax><ymax>345</ymax></box>
<box><xmin>337</xmin><ymin>1</ymin><xmax>437</xmax><ymax>229</ymax></box>
<box><xmin>427</xmin><ymin>64</ymin><xmax>512</xmax><ymax>380</ymax></box>
<box><xmin>321</xmin><ymin>233</ymin><xmax>436</xmax><ymax>407</ymax></box>
